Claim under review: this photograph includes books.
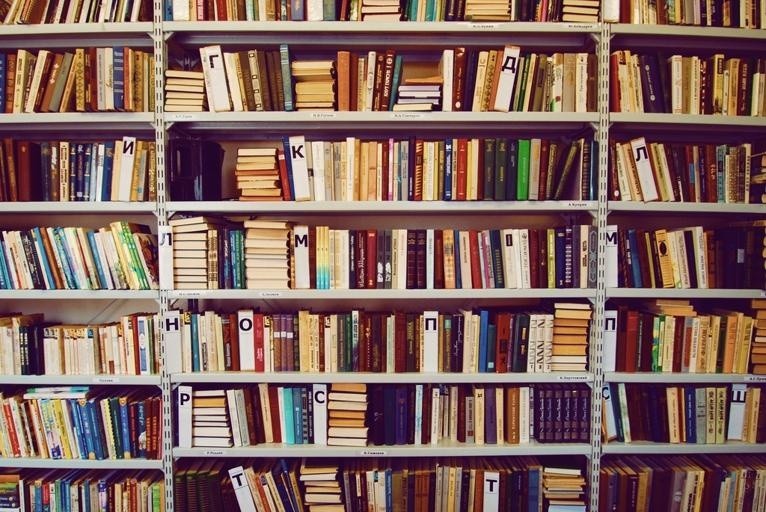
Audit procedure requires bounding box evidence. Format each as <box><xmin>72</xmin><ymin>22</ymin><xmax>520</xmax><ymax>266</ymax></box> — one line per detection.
<box><xmin>161</xmin><ymin>1</ymin><xmax>600</xmax><ymax>512</ymax></box>
<box><xmin>2</xmin><ymin>0</ymin><xmax>164</xmax><ymax>512</ymax></box>
<box><xmin>601</xmin><ymin>1</ymin><xmax>766</xmax><ymax>511</ymax></box>
